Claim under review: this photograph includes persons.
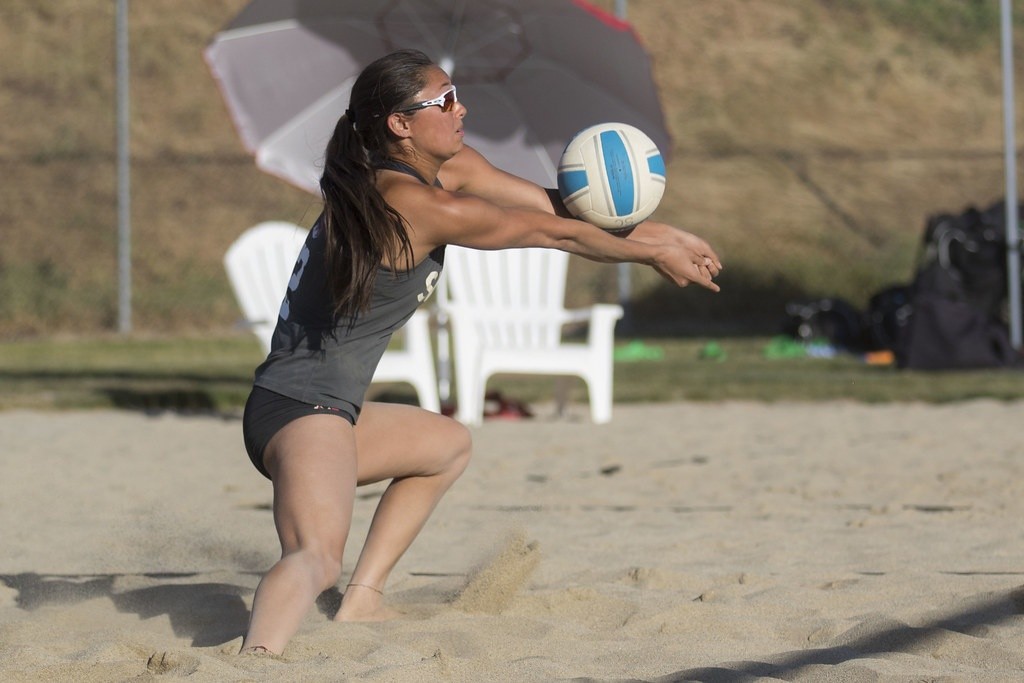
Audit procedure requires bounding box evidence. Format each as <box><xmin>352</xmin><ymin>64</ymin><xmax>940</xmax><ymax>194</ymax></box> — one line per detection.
<box><xmin>224</xmin><ymin>46</ymin><xmax>721</xmax><ymax>672</ymax></box>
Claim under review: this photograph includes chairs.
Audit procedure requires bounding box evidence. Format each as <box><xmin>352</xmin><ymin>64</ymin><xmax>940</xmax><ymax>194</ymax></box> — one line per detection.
<box><xmin>434</xmin><ymin>244</ymin><xmax>625</xmax><ymax>427</ymax></box>
<box><xmin>223</xmin><ymin>220</ymin><xmax>443</xmax><ymax>414</ymax></box>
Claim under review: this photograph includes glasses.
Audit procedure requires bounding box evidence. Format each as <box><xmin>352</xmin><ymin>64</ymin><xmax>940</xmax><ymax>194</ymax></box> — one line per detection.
<box><xmin>391</xmin><ymin>86</ymin><xmax>457</xmax><ymax>112</ymax></box>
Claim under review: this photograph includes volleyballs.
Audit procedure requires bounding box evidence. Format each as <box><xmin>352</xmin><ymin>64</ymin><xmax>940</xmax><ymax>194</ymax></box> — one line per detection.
<box><xmin>556</xmin><ymin>122</ymin><xmax>666</xmax><ymax>232</ymax></box>
